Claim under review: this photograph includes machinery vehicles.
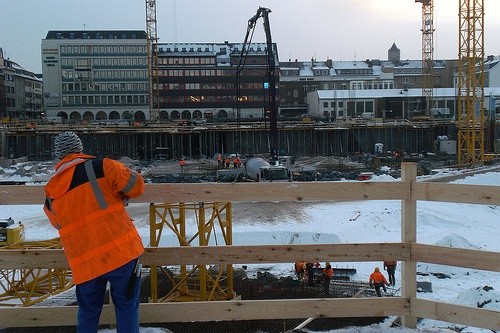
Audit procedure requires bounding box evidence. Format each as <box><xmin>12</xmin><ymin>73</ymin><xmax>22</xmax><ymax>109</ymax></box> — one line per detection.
<box><xmin>243</xmin><ymin>157</ymin><xmax>293</xmax><ymax>182</ymax></box>
<box><xmin>235</xmin><ymin>5</ymin><xmax>283</xmax><ymax>166</ymax></box>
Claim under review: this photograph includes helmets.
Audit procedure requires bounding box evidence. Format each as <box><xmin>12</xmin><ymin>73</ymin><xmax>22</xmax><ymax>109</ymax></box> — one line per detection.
<box><xmin>303</xmin><ymin>265</ymin><xmax>306</xmax><ymax>270</ymax></box>
<box><xmin>326</xmin><ymin>262</ymin><xmax>330</xmax><ymax>266</ymax></box>
<box><xmin>375</xmin><ymin>267</ymin><xmax>379</xmax><ymax>272</ymax></box>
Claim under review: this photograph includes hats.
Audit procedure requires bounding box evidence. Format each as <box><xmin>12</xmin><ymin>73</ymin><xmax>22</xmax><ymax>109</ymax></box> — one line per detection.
<box><xmin>54</xmin><ymin>131</ymin><xmax>83</xmax><ymax>159</ymax></box>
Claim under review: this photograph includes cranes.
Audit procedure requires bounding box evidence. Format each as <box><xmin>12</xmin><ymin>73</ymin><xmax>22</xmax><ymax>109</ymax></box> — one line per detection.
<box><xmin>458</xmin><ymin>0</ymin><xmax>485</xmax><ymax>171</ymax></box>
<box><xmin>415</xmin><ymin>0</ymin><xmax>436</xmax><ymax>116</ymax></box>
<box><xmin>145</xmin><ymin>0</ymin><xmax>160</xmax><ymax>124</ymax></box>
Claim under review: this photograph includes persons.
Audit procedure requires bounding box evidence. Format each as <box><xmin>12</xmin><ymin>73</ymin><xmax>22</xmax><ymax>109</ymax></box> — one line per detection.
<box><xmin>295</xmin><ymin>260</ymin><xmax>316</xmax><ymax>287</ymax></box>
<box><xmin>215</xmin><ymin>153</ymin><xmax>244</xmax><ymax>168</ymax></box>
<box><xmin>179</xmin><ymin>159</ymin><xmax>185</xmax><ymax>169</ymax></box>
<box><xmin>322</xmin><ymin>262</ymin><xmax>333</xmax><ymax>298</ymax></box>
<box><xmin>384</xmin><ymin>259</ymin><xmax>396</xmax><ymax>285</ymax></box>
<box><xmin>43</xmin><ymin>130</ymin><xmax>145</xmax><ymax>333</ymax></box>
<box><xmin>370</xmin><ymin>267</ymin><xmax>390</xmax><ymax>297</ymax></box>
